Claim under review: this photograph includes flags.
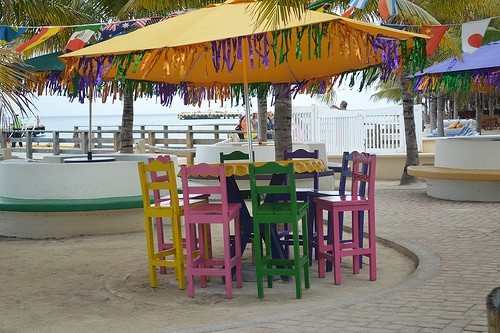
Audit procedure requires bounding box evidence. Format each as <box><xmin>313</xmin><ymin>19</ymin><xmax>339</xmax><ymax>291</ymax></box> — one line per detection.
<box><xmin>12</xmin><ymin>25</ymin><xmax>64</xmax><ymax>52</ymax></box>
<box><xmin>0</xmin><ymin>26</ymin><xmax>28</xmax><ymax>48</ymax></box>
<box><xmin>306</xmin><ymin>0</ymin><xmax>400</xmax><ymax>20</ymax></box>
<box><xmin>96</xmin><ymin>18</ymin><xmax>166</xmax><ymax>44</ymax></box>
<box><xmin>60</xmin><ymin>24</ymin><xmax>103</xmax><ymax>52</ymax></box>
<box><xmin>379</xmin><ymin>23</ymin><xmax>406</xmax><ymax>31</ymax></box>
<box><xmin>462</xmin><ymin>19</ymin><xmax>491</xmax><ymax>53</ymax></box>
<box><xmin>418</xmin><ymin>25</ymin><xmax>450</xmax><ymax>56</ymax></box>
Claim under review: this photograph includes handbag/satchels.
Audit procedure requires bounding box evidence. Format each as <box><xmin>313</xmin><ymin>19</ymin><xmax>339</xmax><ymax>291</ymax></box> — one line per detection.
<box><xmin>231</xmin><ymin>132</ymin><xmax>240</xmax><ymax>142</ymax></box>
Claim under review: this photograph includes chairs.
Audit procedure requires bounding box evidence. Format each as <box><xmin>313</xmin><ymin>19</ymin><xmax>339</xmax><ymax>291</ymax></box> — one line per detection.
<box><xmin>308</xmin><ymin>151</ymin><xmax>370</xmax><ymax>274</ymax></box>
<box><xmin>311</xmin><ymin>151</ymin><xmax>376</xmax><ymax>287</ymax></box>
<box><xmin>218</xmin><ymin>148</ymin><xmax>258</xmax><ymax>259</ymax></box>
<box><xmin>149</xmin><ymin>154</ymin><xmax>215</xmax><ymax>275</ymax></box>
<box><xmin>135</xmin><ymin>158</ymin><xmax>208</xmax><ymax>294</ymax></box>
<box><xmin>281</xmin><ymin>148</ymin><xmax>323</xmax><ymax>268</ymax></box>
<box><xmin>248</xmin><ymin>160</ymin><xmax>311</xmax><ymax>301</ymax></box>
<box><xmin>181</xmin><ymin>162</ymin><xmax>242</xmax><ymax>300</ymax></box>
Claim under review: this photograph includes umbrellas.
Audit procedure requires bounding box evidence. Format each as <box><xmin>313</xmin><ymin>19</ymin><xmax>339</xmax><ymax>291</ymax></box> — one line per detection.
<box><xmin>404</xmin><ymin>40</ymin><xmax>500</xmax><ymax>79</ymax></box>
<box><xmin>59</xmin><ymin>0</ymin><xmax>431</xmax><ymax>161</ymax></box>
<box><xmin>9</xmin><ymin>45</ymin><xmax>95</xmax><ymax>163</ymax></box>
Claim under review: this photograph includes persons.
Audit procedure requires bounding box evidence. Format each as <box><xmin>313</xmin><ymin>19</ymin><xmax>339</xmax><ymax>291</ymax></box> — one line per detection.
<box><xmin>236</xmin><ymin>113</ymin><xmax>258</xmax><ymax>140</ymax></box>
<box><xmin>12</xmin><ymin>115</ymin><xmax>22</xmax><ymax>147</ymax></box>
<box><xmin>266</xmin><ymin>117</ymin><xmax>275</xmax><ymax>139</ymax></box>
<box><xmin>331</xmin><ymin>100</ymin><xmax>347</xmax><ymax>111</ymax></box>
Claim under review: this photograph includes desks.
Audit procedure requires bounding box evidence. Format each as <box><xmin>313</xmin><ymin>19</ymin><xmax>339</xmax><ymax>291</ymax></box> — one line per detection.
<box><xmin>171</xmin><ymin>156</ymin><xmax>332</xmax><ymax>284</ymax></box>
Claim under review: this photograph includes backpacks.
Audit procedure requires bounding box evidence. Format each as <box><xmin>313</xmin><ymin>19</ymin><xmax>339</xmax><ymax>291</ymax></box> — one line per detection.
<box><xmin>235</xmin><ymin>125</ymin><xmax>245</xmax><ymax>139</ymax></box>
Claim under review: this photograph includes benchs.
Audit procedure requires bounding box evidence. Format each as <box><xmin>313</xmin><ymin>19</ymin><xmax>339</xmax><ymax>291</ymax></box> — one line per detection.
<box><xmin>0</xmin><ymin>193</ymin><xmax>164</xmax><ymax>214</ymax></box>
<box><xmin>326</xmin><ymin>161</ymin><xmax>353</xmax><ymax>173</ymax></box>
<box><xmin>403</xmin><ymin>162</ymin><xmax>500</xmax><ymax>182</ymax></box>
